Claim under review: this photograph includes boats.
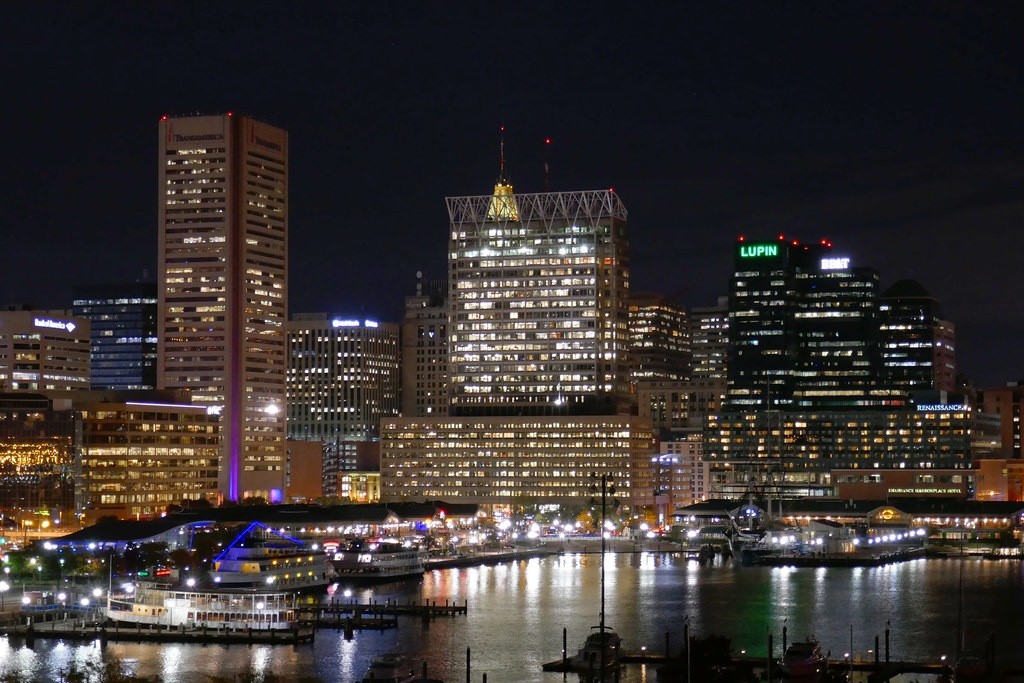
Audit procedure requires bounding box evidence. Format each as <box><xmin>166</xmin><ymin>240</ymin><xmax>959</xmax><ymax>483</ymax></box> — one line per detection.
<box><xmin>106</xmin><ymin>580</ymin><xmax>301</xmax><ymax>633</ymax></box>
<box><xmin>207</xmin><ymin>546</ymin><xmax>331</xmax><ymax>591</ymax></box>
<box><xmin>777</xmin><ymin>640</ymin><xmax>826</xmax><ymax>677</ymax></box>
<box><xmin>955</xmin><ymin>647</ymin><xmax>989</xmax><ymax>678</ymax></box>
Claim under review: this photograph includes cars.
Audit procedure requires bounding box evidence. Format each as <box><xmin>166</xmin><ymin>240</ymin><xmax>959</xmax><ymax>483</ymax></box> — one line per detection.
<box><xmin>329</xmin><ymin>541</ymin><xmax>426</xmax><ymax>582</ymax></box>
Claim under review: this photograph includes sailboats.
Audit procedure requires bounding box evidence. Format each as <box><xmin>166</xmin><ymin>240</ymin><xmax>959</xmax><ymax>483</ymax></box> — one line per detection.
<box><xmin>572</xmin><ymin>471</ymin><xmax>623</xmax><ymax>680</ymax></box>
